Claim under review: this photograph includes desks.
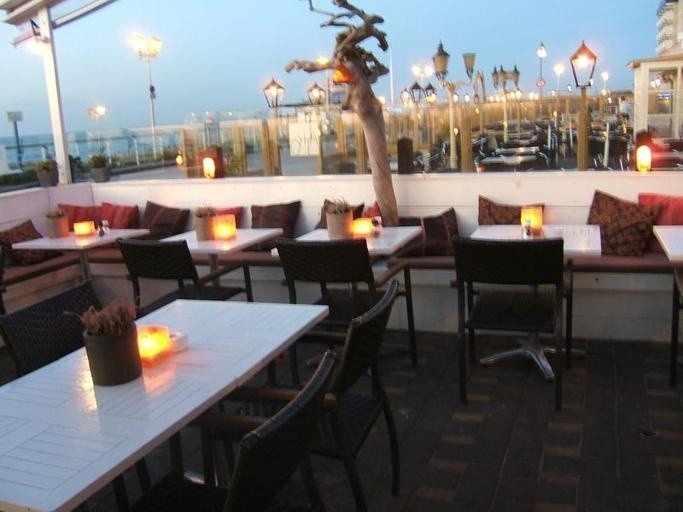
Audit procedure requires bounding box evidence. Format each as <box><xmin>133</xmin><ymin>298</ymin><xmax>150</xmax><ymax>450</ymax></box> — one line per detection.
<box><xmin>398</xmin><ymin>124</ymin><xmax>681</xmax><ymax>171</ymax></box>
<box><xmin>26</xmin><ymin>226</ymin><xmax>150</xmax><ymax>330</ymax></box>
<box><xmin>272</xmin><ymin>230</ymin><xmax>420</xmax><ymax>379</ymax></box>
<box><xmin>154</xmin><ymin>222</ymin><xmax>284</xmax><ymax>299</ymax></box>
<box><xmin>467</xmin><ymin>222</ymin><xmax>682</xmax><ymax>394</ymax></box>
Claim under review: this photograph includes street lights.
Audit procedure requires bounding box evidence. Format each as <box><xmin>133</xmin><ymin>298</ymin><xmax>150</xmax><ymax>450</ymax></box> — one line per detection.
<box><xmin>305</xmin><ymin>85</ymin><xmax>326</xmax><ymax>173</ymax></box>
<box><xmin>263</xmin><ymin>77</ymin><xmax>286</xmax><ymax>176</ymax></box>
<box><xmin>88</xmin><ymin>105</ymin><xmax>109</xmax><ymax>155</ymax></box>
<box><xmin>316</xmin><ymin>56</ymin><xmax>329</xmax><ymax>85</ymax></box>
<box><xmin>126</xmin><ymin>32</ymin><xmax>163</xmax><ymax>158</ymax></box>
<box><xmin>373</xmin><ymin>40</ymin><xmax>616</xmax><ymax>172</ymax></box>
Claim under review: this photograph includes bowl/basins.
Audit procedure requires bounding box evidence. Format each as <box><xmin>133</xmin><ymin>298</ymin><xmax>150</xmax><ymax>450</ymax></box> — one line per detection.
<box><xmin>541</xmin><ymin>223</ymin><xmax>565</xmax><ymax>240</ymax></box>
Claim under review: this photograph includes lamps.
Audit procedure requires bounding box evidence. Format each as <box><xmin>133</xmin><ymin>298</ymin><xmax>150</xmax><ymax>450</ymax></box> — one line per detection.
<box><xmin>73</xmin><ymin>219</ymin><xmax>93</xmax><ymax>235</ymax></box>
<box><xmin>134</xmin><ymin>325</ymin><xmax>173</xmax><ymax>361</ymax></box>
<box><xmin>520</xmin><ymin>204</ymin><xmax>542</xmax><ymax>235</ymax></box>
<box><xmin>199</xmin><ymin>146</ymin><xmax>220</xmax><ymax>178</ymax></box>
<box><xmin>213</xmin><ymin>214</ymin><xmax>236</xmax><ymax>240</ymax></box>
<box><xmin>348</xmin><ymin>216</ymin><xmax>373</xmax><ymax>236</ymax></box>
<box><xmin>4</xmin><ymin>108</ymin><xmax>24</xmax><ymax>122</ymax></box>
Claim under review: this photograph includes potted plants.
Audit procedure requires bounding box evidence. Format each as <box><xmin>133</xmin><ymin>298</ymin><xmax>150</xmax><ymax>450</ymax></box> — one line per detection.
<box><xmin>324</xmin><ymin>200</ymin><xmax>353</xmax><ymax>239</ymax></box>
<box><xmin>43</xmin><ymin>209</ymin><xmax>64</xmax><ymax>237</ymax></box>
<box><xmin>78</xmin><ymin>303</ymin><xmax>140</xmax><ymax>390</ymax></box>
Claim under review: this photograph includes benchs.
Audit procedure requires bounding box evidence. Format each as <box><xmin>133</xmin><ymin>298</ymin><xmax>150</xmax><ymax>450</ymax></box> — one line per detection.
<box><xmin>1</xmin><ymin>238</ymin><xmax>670</xmax><ymax>345</ymax></box>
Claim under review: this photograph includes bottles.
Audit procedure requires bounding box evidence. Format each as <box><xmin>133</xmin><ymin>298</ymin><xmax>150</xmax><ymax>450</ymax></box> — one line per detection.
<box><xmin>521</xmin><ymin>219</ymin><xmax>534</xmax><ymax>240</ymax></box>
<box><xmin>370</xmin><ymin>215</ymin><xmax>384</xmax><ymax>239</ymax></box>
<box><xmin>97</xmin><ymin>220</ymin><xmax>110</xmax><ymax>236</ymax></box>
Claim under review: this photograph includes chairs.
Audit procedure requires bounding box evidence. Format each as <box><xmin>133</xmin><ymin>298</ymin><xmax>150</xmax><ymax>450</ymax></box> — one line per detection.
<box><xmin>136</xmin><ymin>355</ymin><xmax>335</xmax><ymax>510</ymax></box>
<box><xmin>226</xmin><ymin>279</ymin><xmax>407</xmax><ymax>510</ymax></box>
<box><xmin>450</xmin><ymin>237</ymin><xmax>569</xmax><ymax>411</ymax></box>
<box><xmin>1</xmin><ymin>283</ymin><xmax>103</xmax><ymax>373</ymax></box>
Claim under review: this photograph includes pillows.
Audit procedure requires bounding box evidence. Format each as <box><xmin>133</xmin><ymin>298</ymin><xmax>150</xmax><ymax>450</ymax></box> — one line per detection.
<box><xmin>2</xmin><ymin>223</ymin><xmax>59</xmax><ymax>263</ymax></box>
<box><xmin>191</xmin><ymin>207</ymin><xmax>245</xmax><ymax>233</ymax></box>
<box><xmin>96</xmin><ymin>199</ymin><xmax>137</xmax><ymax>230</ymax></box>
<box><xmin>139</xmin><ymin>199</ymin><xmax>188</xmax><ymax>239</ymax></box>
<box><xmin>639</xmin><ymin>191</ymin><xmax>683</xmax><ymax>226</ymax></box>
<box><xmin>395</xmin><ymin>208</ymin><xmax>462</xmax><ymax>252</ymax></box>
<box><xmin>58</xmin><ymin>203</ymin><xmax>98</xmax><ymax>229</ymax></box>
<box><xmin>583</xmin><ymin>191</ymin><xmax>663</xmax><ymax>258</ymax></box>
<box><xmin>476</xmin><ymin>197</ymin><xmax>520</xmax><ymax>223</ymax></box>
<box><xmin>250</xmin><ymin>200</ymin><xmax>300</xmax><ymax>239</ymax></box>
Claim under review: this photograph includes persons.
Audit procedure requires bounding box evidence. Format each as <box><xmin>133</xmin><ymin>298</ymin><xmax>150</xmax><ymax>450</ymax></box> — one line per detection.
<box><xmin>523</xmin><ymin>120</ymin><xmax>547</xmax><ymax>150</ymax></box>
<box><xmin>618</xmin><ymin>94</ymin><xmax>632</xmax><ymax>127</ymax></box>
